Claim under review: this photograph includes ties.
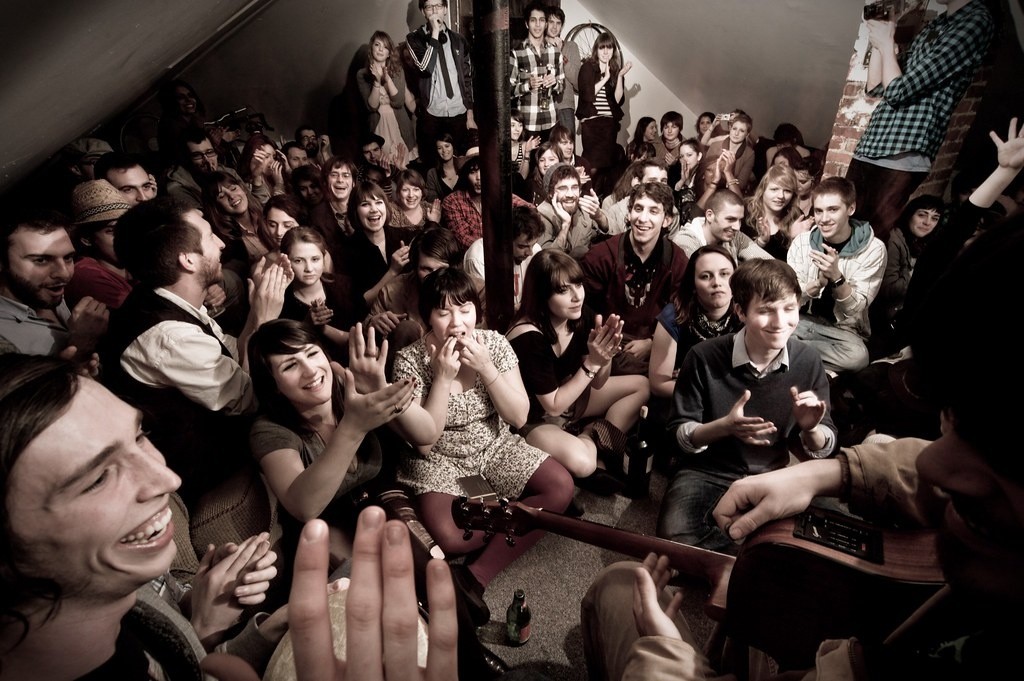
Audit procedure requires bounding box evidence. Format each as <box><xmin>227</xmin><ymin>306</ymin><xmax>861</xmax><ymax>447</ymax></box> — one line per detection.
<box><xmin>437</xmin><ymin>43</ymin><xmax>454</xmax><ymax>100</ymax></box>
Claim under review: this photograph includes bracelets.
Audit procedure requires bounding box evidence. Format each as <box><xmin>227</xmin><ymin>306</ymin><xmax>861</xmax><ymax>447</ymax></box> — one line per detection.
<box><xmin>726</xmin><ymin>179</ymin><xmax>740</xmax><ymax>188</ymax></box>
<box><xmin>711</xmin><ymin>181</ymin><xmax>718</xmax><ymax>186</ymax></box>
<box><xmin>486</xmin><ymin>372</ymin><xmax>499</xmax><ymax>389</ymax></box>
<box><xmin>374</xmin><ymin>85</ymin><xmax>380</xmax><ymax>88</ymax></box>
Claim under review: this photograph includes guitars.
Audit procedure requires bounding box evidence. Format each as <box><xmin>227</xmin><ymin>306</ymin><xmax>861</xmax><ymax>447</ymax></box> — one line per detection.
<box><xmin>450</xmin><ymin>495</ymin><xmax>951</xmax><ymax>674</ymax></box>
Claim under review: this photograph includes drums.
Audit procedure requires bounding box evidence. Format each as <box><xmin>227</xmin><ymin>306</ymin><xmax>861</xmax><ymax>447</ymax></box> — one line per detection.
<box><xmin>261</xmin><ymin>581</ymin><xmax>430</xmax><ymax>681</ymax></box>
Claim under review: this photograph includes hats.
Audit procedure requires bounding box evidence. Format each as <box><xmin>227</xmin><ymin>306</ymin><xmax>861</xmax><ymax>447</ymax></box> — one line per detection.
<box><xmin>543</xmin><ymin>162</ymin><xmax>568</xmax><ymax>194</ymax></box>
<box><xmin>353</xmin><ymin>133</ymin><xmax>385</xmax><ymax>149</ymax></box>
<box><xmin>70</xmin><ymin>138</ymin><xmax>114</xmax><ymax>162</ymax></box>
<box><xmin>457</xmin><ymin>146</ymin><xmax>479</xmax><ymax>169</ymax></box>
<box><xmin>68</xmin><ymin>178</ymin><xmax>132</xmax><ymax>225</ymax></box>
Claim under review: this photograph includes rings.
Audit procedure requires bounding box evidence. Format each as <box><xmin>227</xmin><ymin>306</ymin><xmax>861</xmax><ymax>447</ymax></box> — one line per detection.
<box><xmin>394</xmin><ymin>405</ymin><xmax>403</xmax><ymax>413</ymax></box>
<box><xmin>611</xmin><ymin>350</ymin><xmax>616</xmax><ymax>356</ymax></box>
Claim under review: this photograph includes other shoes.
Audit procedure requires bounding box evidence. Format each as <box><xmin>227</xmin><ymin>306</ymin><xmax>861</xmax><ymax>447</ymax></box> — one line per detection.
<box><xmin>451</xmin><ymin>565</ymin><xmax>490</xmax><ymax>627</ymax></box>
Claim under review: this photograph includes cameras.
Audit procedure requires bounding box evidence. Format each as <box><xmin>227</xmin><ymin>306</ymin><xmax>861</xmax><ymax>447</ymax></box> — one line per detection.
<box><xmin>863</xmin><ymin>0</ymin><xmax>905</xmax><ymax>21</ymax></box>
<box><xmin>721</xmin><ymin>114</ymin><xmax>730</xmax><ymax>120</ymax></box>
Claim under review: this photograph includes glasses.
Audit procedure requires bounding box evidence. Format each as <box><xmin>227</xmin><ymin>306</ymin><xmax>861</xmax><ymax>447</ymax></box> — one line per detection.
<box><xmin>189</xmin><ymin>151</ymin><xmax>216</xmax><ymax>160</ymax></box>
<box><xmin>424</xmin><ymin>4</ymin><xmax>444</xmax><ymax>10</ymax></box>
<box><xmin>83</xmin><ymin>160</ymin><xmax>97</xmax><ymax>168</ymax></box>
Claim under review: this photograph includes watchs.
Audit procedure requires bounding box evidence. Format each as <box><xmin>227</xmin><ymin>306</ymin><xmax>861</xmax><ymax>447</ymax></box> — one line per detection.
<box><xmin>581</xmin><ymin>362</ymin><xmax>597</xmax><ymax>379</ymax></box>
<box><xmin>831</xmin><ymin>274</ymin><xmax>846</xmax><ymax>287</ymax></box>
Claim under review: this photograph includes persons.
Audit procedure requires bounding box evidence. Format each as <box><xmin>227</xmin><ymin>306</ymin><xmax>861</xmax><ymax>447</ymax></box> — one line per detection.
<box><xmin>0</xmin><ymin>0</ymin><xmax>1024</xmax><ymax>681</ymax></box>
<box><xmin>392</xmin><ymin>264</ymin><xmax>575</xmax><ymax>548</ymax></box>
<box><xmin>356</xmin><ymin>30</ymin><xmax>420</xmax><ymax>172</ymax></box>
<box><xmin>667</xmin><ymin>258</ymin><xmax>840</xmax><ymax>546</ymax></box>
<box><xmin>406</xmin><ymin>0</ymin><xmax>478</xmax><ymax>170</ymax></box>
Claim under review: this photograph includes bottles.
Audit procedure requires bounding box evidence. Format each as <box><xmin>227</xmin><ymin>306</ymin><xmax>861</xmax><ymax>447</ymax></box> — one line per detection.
<box><xmin>507</xmin><ymin>589</ymin><xmax>532</xmax><ymax>647</ymax></box>
<box><xmin>621</xmin><ymin>406</ymin><xmax>655</xmax><ymax>499</ymax></box>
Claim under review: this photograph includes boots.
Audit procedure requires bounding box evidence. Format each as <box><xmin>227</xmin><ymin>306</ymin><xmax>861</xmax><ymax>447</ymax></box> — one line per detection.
<box><xmin>572</xmin><ymin>418</ymin><xmax>628</xmax><ymax>495</ymax></box>
